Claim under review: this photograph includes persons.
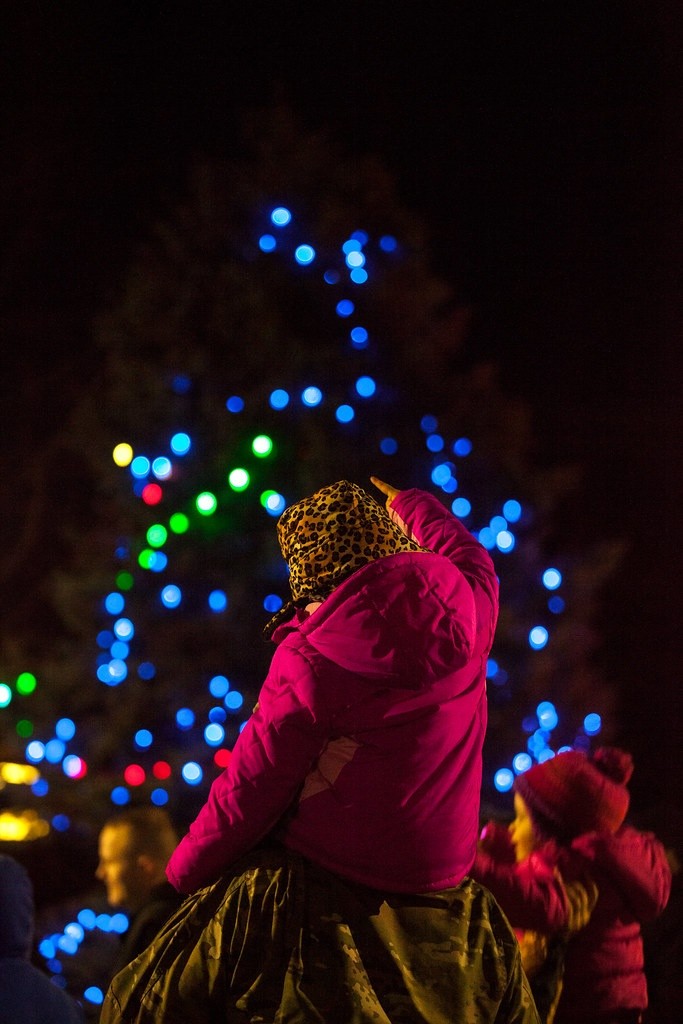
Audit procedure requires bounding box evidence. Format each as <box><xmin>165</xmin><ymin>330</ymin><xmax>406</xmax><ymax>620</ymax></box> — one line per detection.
<box><xmin>468</xmin><ymin>746</ymin><xmax>676</xmax><ymax>1024</ymax></box>
<box><xmin>100</xmin><ymin>860</ymin><xmax>542</xmax><ymax>1024</ymax></box>
<box><xmin>0</xmin><ymin>855</ymin><xmax>85</xmax><ymax>1024</ymax></box>
<box><xmin>167</xmin><ymin>477</ymin><xmax>500</xmax><ymax>894</ymax></box>
<box><xmin>96</xmin><ymin>810</ymin><xmax>188</xmax><ymax>972</ymax></box>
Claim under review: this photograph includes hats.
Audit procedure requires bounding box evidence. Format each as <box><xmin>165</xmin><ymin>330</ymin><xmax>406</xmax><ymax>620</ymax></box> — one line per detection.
<box><xmin>513</xmin><ymin>743</ymin><xmax>633</xmax><ymax>838</ymax></box>
<box><xmin>262</xmin><ymin>481</ymin><xmax>433</xmax><ymax>639</ymax></box>
